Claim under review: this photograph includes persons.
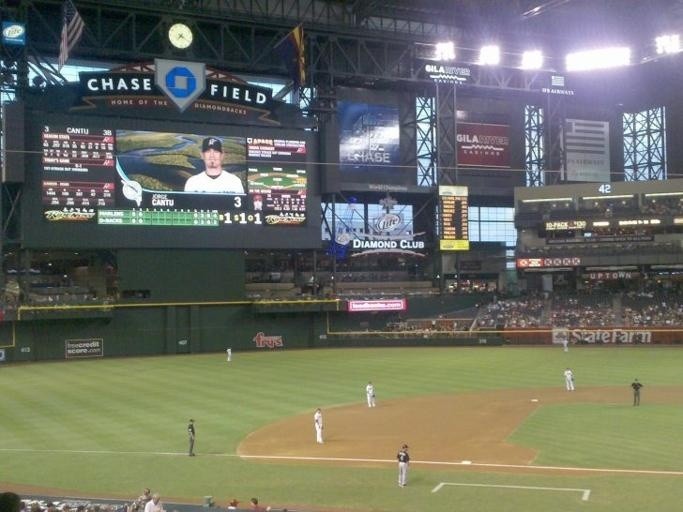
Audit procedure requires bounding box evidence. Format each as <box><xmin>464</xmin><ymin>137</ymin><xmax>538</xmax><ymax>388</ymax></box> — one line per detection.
<box><xmin>0</xmin><ymin>488</ymin><xmax>167</xmax><ymax>512</ymax></box>
<box><xmin>632</xmin><ymin>378</ymin><xmax>643</xmax><ymax>406</ymax></box>
<box><xmin>397</xmin><ymin>444</ymin><xmax>410</xmax><ymax>486</ymax></box>
<box><xmin>226</xmin><ymin>347</ymin><xmax>231</xmax><ymax>362</ymax></box>
<box><xmin>366</xmin><ymin>381</ymin><xmax>375</xmax><ymax>407</ymax></box>
<box><xmin>228</xmin><ymin>498</ymin><xmax>271</xmax><ymax>512</ymax></box>
<box><xmin>562</xmin><ymin>336</ymin><xmax>569</xmax><ymax>351</ymax></box>
<box><xmin>407</xmin><ymin>280</ymin><xmax>683</xmax><ymax>331</ymax></box>
<box><xmin>188</xmin><ymin>419</ymin><xmax>195</xmax><ymax>456</ymax></box>
<box><xmin>184</xmin><ymin>138</ymin><xmax>245</xmax><ymax>193</ymax></box>
<box><xmin>564</xmin><ymin>368</ymin><xmax>574</xmax><ymax>391</ymax></box>
<box><xmin>314</xmin><ymin>408</ymin><xmax>324</xmax><ymax>445</ymax></box>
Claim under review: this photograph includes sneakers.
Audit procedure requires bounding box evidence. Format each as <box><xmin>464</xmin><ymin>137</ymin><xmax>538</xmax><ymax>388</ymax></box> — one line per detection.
<box><xmin>369</xmin><ymin>404</ymin><xmax>375</xmax><ymax>407</ymax></box>
<box><xmin>399</xmin><ymin>485</ymin><xmax>406</xmax><ymax>488</ymax></box>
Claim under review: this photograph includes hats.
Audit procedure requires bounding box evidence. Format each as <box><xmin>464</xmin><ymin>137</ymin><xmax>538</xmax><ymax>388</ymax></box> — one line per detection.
<box><xmin>202</xmin><ymin>137</ymin><xmax>222</xmax><ymax>153</ymax></box>
